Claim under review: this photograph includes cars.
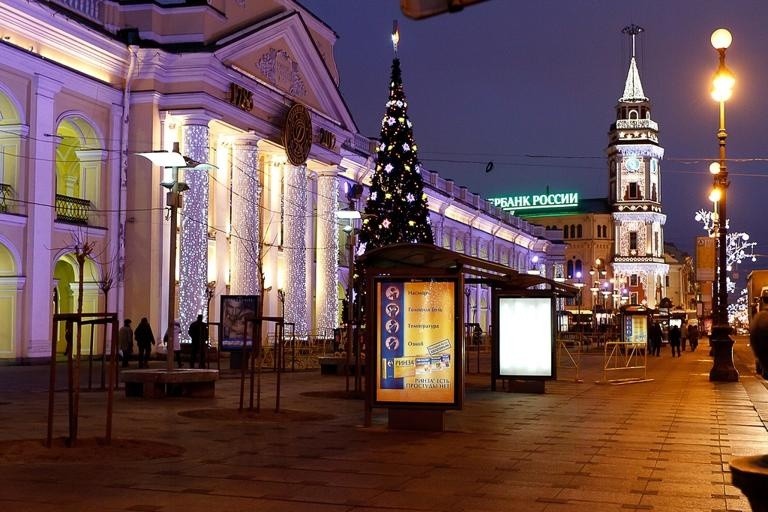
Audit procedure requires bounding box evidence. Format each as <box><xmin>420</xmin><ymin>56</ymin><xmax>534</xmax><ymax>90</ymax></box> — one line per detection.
<box><xmin>558</xmin><ymin>307</ymin><xmax>750</xmax><ymax>343</ymax></box>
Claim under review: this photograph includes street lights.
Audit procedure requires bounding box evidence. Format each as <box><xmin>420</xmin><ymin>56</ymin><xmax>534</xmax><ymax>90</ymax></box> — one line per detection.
<box><xmin>705</xmin><ymin>25</ymin><xmax>742</xmax><ymax>385</ymax></box>
<box><xmin>704</xmin><ymin>159</ymin><xmax>724</xmax><ymax>352</ymax></box>
<box><xmin>464</xmin><ymin>287</ymin><xmax>472</xmax><ymax>341</ymax></box>
<box><xmin>531</xmin><ymin>256</ymin><xmax>539</xmax><ymax>289</ymax></box>
<box><xmin>132</xmin><ymin>140</ymin><xmax>219</xmax><ymax>371</ymax></box>
<box><xmin>334</xmin><ymin>179</ymin><xmax>378</xmax><ymax>360</ymax></box>
<box><xmin>572</xmin><ymin>271</ymin><xmax>587</xmax><ymax>337</ymax></box>
<box><xmin>589</xmin><ymin>258</ymin><xmax>632</xmax><ymax>333</ymax></box>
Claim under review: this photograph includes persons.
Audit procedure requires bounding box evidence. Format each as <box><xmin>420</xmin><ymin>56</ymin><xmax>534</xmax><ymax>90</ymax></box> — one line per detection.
<box><xmin>163</xmin><ymin>322</ymin><xmax>184</xmax><ymax>367</ymax></box>
<box><xmin>649</xmin><ymin>322</ymin><xmax>698</xmax><ymax>358</ymax></box>
<box><xmin>473</xmin><ymin>325</ymin><xmax>483</xmax><ymax>344</ymax></box>
<box><xmin>224</xmin><ymin>299</ymin><xmax>255</xmax><ymax>339</ymax></box>
<box><xmin>188</xmin><ymin>315</ymin><xmax>208</xmax><ymax>369</ymax></box>
<box><xmin>120</xmin><ymin>319</ymin><xmax>133</xmax><ymax>367</ymax></box>
<box><xmin>134</xmin><ymin>317</ymin><xmax>155</xmax><ymax>369</ymax></box>
